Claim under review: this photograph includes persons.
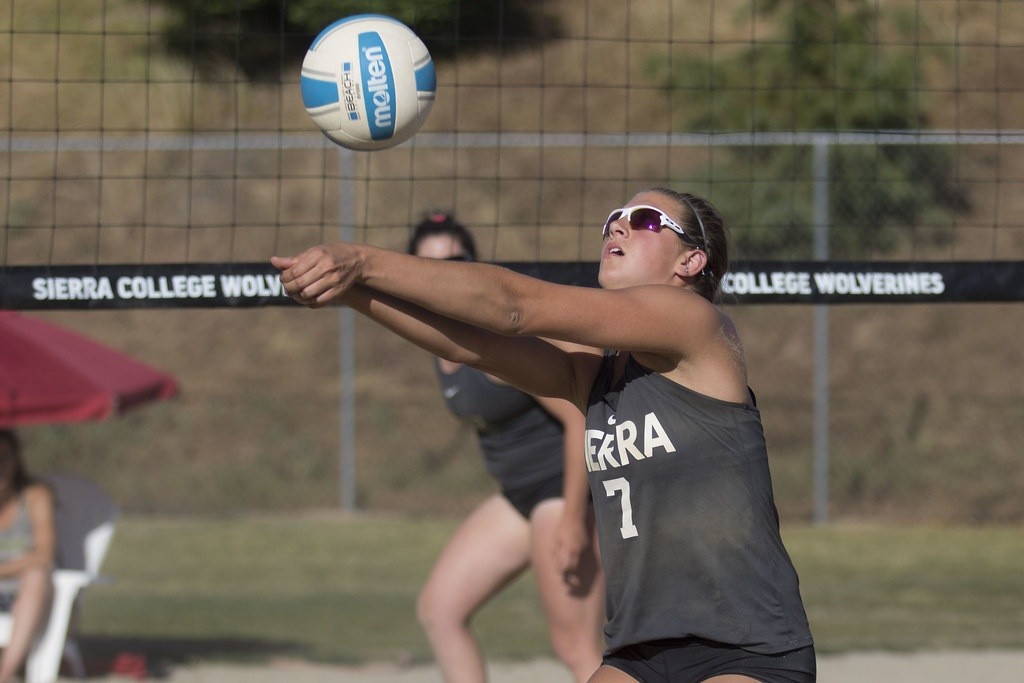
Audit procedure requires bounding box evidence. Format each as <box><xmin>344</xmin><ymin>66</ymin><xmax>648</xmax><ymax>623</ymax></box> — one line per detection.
<box><xmin>0</xmin><ymin>427</ymin><xmax>62</xmax><ymax>683</ymax></box>
<box><xmin>403</xmin><ymin>209</ymin><xmax>610</xmax><ymax>683</ymax></box>
<box><xmin>271</xmin><ymin>188</ymin><xmax>816</xmax><ymax>683</ymax></box>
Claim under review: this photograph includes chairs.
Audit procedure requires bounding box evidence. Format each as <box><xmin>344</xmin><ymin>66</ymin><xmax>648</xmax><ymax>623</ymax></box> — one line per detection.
<box><xmin>0</xmin><ymin>466</ymin><xmax>120</xmax><ymax>683</ymax></box>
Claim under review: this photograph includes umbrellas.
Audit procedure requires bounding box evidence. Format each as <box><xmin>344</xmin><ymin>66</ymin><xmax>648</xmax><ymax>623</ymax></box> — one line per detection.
<box><xmin>0</xmin><ymin>309</ymin><xmax>180</xmax><ymax>427</ymax></box>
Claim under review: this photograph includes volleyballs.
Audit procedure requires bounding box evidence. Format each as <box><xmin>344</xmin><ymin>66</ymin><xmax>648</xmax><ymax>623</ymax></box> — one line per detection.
<box><xmin>299</xmin><ymin>13</ymin><xmax>440</xmax><ymax>155</ymax></box>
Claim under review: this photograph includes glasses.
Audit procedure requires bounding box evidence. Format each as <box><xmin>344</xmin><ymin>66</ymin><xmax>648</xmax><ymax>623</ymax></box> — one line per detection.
<box><xmin>602</xmin><ymin>205</ymin><xmax>704</xmax><ymax>253</ymax></box>
